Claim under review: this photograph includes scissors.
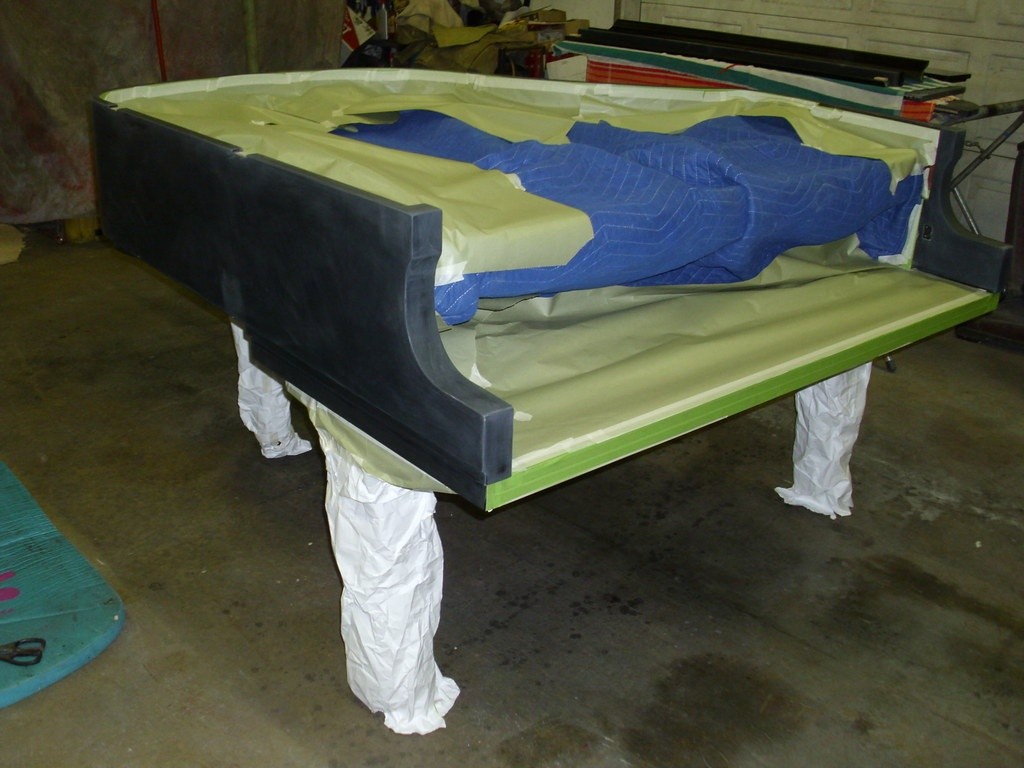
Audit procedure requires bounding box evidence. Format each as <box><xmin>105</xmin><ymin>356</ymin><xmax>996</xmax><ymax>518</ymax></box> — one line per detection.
<box><xmin>0</xmin><ymin>637</ymin><xmax>46</xmax><ymax>666</ymax></box>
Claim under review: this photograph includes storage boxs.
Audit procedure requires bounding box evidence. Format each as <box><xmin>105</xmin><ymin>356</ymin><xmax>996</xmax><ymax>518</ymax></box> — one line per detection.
<box><xmin>538</xmin><ymin>9</ymin><xmax>566</xmax><ymax>22</ymax></box>
<box><xmin>529</xmin><ymin>19</ymin><xmax>589</xmax><ymax>37</ymax></box>
